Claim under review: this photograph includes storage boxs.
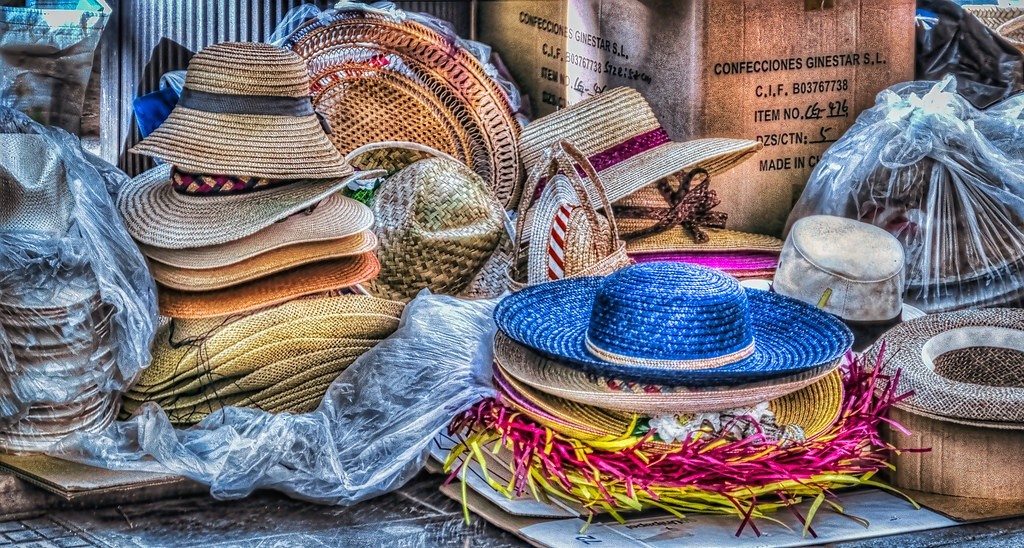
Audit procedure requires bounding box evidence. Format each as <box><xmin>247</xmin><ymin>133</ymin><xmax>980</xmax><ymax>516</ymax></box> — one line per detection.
<box><xmin>480</xmin><ymin>0</ymin><xmax>916</xmax><ymax>233</ymax></box>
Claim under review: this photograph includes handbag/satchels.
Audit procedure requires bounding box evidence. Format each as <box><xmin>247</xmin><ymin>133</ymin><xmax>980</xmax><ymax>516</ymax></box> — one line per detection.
<box><xmin>504</xmin><ymin>139</ymin><xmax>631</xmax><ymax>293</ymax></box>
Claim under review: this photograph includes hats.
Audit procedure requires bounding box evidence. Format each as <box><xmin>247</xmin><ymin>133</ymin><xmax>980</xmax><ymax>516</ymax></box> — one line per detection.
<box><xmin>739</xmin><ymin>214</ymin><xmax>928</xmax><ymax>367</ymax></box>
<box><xmin>0</xmin><ymin>40</ymin><xmax>854</xmax><ymax>456</ymax></box>
<box><xmin>861</xmin><ymin>304</ymin><xmax>1024</xmax><ymax>427</ymax></box>
